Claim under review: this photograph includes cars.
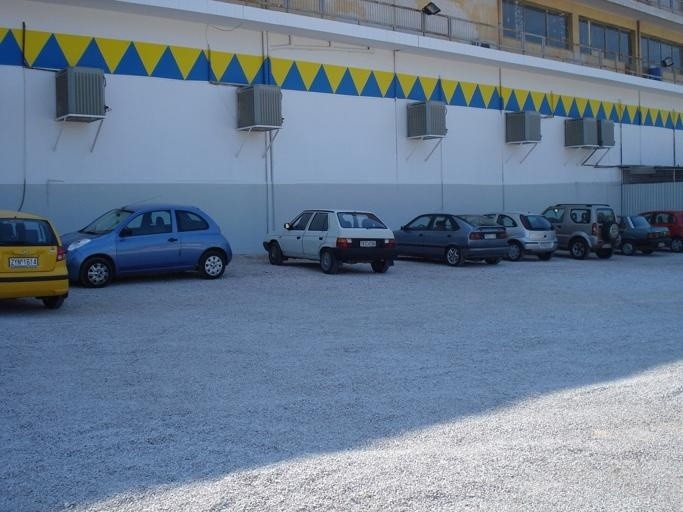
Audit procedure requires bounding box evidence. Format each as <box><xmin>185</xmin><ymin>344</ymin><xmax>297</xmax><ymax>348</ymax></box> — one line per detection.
<box><xmin>392</xmin><ymin>200</ymin><xmax>683</xmax><ymax>267</ymax></box>
<box><xmin>0</xmin><ymin>208</ymin><xmax>72</xmax><ymax>313</ymax></box>
<box><xmin>261</xmin><ymin>206</ymin><xmax>399</xmax><ymax>279</ymax></box>
<box><xmin>57</xmin><ymin>196</ymin><xmax>234</xmax><ymax>287</ymax></box>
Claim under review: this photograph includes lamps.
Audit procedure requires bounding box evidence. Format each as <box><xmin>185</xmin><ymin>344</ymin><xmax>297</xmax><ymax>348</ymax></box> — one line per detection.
<box><xmin>422</xmin><ymin>2</ymin><xmax>441</xmax><ymax>15</ymax></box>
<box><xmin>663</xmin><ymin>56</ymin><xmax>674</xmax><ymax>67</ymax></box>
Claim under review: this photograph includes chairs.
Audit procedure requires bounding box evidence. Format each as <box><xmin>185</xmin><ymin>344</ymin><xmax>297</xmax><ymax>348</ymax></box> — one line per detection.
<box><xmin>2</xmin><ymin>224</ymin><xmax>39</xmax><ymax>244</ymax></box>
<box><xmin>433</xmin><ymin>221</ymin><xmax>446</xmax><ymax>230</ymax></box>
<box><xmin>152</xmin><ymin>217</ymin><xmax>166</xmax><ymax>234</ymax></box>
<box><xmin>571</xmin><ymin>212</ymin><xmax>589</xmax><ymax>224</ymax></box>
<box><xmin>341</xmin><ymin>219</ymin><xmax>375</xmax><ymax>228</ymax></box>
<box><xmin>657</xmin><ymin>215</ymin><xmax>673</xmax><ymax>223</ymax></box>
